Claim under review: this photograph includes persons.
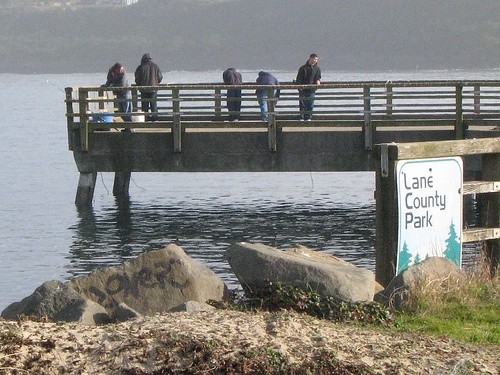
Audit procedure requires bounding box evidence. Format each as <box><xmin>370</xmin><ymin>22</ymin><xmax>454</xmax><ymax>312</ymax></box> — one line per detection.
<box><xmin>295</xmin><ymin>53</ymin><xmax>321</xmax><ymax>121</ymax></box>
<box><xmin>255</xmin><ymin>71</ymin><xmax>281</xmax><ymax>121</ymax></box>
<box><xmin>134</xmin><ymin>52</ymin><xmax>163</xmax><ymax>122</ymax></box>
<box><xmin>100</xmin><ymin>62</ymin><xmax>133</xmax><ymax>132</ymax></box>
<box><xmin>223</xmin><ymin>67</ymin><xmax>242</xmax><ymax>122</ymax></box>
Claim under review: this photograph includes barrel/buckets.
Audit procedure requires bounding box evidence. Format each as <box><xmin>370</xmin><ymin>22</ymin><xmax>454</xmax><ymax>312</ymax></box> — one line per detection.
<box><xmin>91</xmin><ymin>112</ymin><xmax>114</xmax><ymax>131</ymax></box>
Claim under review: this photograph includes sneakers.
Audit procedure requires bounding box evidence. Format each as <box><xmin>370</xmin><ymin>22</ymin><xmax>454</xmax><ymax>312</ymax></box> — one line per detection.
<box><xmin>307</xmin><ymin>118</ymin><xmax>311</xmax><ymax>122</ymax></box>
<box><xmin>300</xmin><ymin>118</ymin><xmax>304</xmax><ymax>121</ymax></box>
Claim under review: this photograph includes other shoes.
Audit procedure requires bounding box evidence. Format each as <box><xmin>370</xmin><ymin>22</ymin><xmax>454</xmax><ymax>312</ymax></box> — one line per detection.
<box><xmin>121</xmin><ymin>128</ymin><xmax>135</xmax><ymax>133</ymax></box>
